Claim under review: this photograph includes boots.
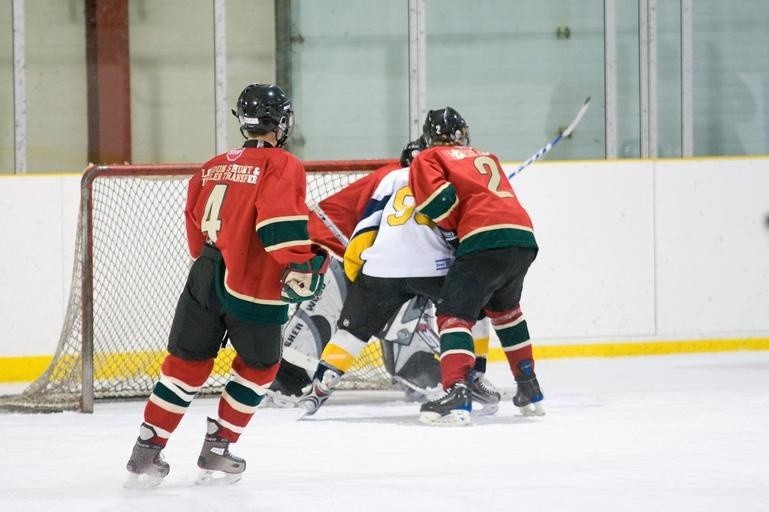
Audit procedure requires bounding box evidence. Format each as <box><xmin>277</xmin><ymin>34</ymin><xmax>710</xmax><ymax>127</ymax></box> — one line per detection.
<box><xmin>421</xmin><ymin>378</ymin><xmax>471</xmax><ymax>414</ymax></box>
<box><xmin>127</xmin><ymin>423</ymin><xmax>170</xmax><ymax>477</ymax></box>
<box><xmin>468</xmin><ymin>357</ymin><xmax>501</xmax><ymax>404</ymax></box>
<box><xmin>299</xmin><ymin>362</ymin><xmax>344</xmax><ymax>415</ymax></box>
<box><xmin>198</xmin><ymin>417</ymin><xmax>246</xmax><ymax>473</ymax></box>
<box><xmin>513</xmin><ymin>360</ymin><xmax>543</xmax><ymax>406</ymax></box>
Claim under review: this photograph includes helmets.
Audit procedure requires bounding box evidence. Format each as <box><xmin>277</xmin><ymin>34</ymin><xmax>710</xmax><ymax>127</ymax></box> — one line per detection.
<box><xmin>232</xmin><ymin>84</ymin><xmax>293</xmax><ymax>143</ymax></box>
<box><xmin>400</xmin><ymin>107</ymin><xmax>470</xmax><ymax>168</ymax></box>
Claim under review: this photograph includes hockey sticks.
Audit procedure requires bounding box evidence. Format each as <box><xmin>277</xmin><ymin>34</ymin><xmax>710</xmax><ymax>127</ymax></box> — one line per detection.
<box><xmin>507</xmin><ymin>95</ymin><xmax>594</xmax><ymax>183</ymax></box>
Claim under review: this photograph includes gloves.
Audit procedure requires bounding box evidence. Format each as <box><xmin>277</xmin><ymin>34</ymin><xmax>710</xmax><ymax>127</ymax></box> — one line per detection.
<box><xmin>279</xmin><ymin>244</ymin><xmax>330</xmax><ymax>303</ymax></box>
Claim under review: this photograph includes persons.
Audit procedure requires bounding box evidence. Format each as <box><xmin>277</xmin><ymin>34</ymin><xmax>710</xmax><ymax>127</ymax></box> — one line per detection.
<box><xmin>258</xmin><ymin>162</ymin><xmax>445</xmax><ymax>393</ymax></box>
<box><xmin>408</xmin><ymin>108</ymin><xmax>547</xmax><ymax>416</ymax></box>
<box><xmin>127</xmin><ymin>84</ymin><xmax>334</xmax><ymax>477</ymax></box>
<box><xmin>299</xmin><ymin>140</ymin><xmax>502</xmax><ymax>412</ymax></box>
